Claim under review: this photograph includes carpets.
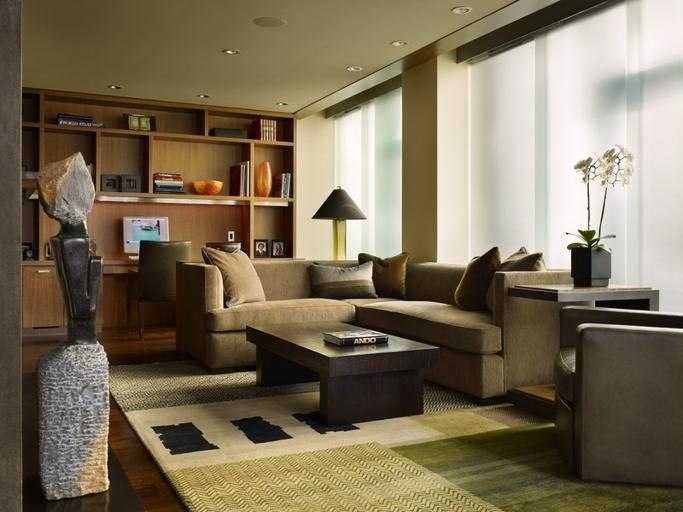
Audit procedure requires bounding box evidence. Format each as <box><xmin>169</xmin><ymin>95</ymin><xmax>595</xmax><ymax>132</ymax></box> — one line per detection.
<box><xmin>108</xmin><ymin>357</ymin><xmax>683</xmax><ymax>511</ymax></box>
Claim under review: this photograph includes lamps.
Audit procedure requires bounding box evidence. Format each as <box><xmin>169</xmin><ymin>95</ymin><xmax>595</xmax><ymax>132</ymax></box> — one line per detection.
<box><xmin>311</xmin><ymin>188</ymin><xmax>369</xmax><ymax>261</ymax></box>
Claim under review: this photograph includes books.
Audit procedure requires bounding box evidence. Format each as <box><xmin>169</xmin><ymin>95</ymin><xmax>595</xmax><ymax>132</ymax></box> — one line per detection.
<box><xmin>57</xmin><ymin>113</ymin><xmax>92</xmax><ymax>122</ymax></box>
<box><xmin>59</xmin><ymin>119</ymin><xmax>104</xmax><ymax>127</ymax></box>
<box><xmin>152</xmin><ymin>118</ymin><xmax>291</xmax><ymax>198</ymax></box>
<box><xmin>321</xmin><ymin>329</ymin><xmax>387</xmax><ymax>346</ymax></box>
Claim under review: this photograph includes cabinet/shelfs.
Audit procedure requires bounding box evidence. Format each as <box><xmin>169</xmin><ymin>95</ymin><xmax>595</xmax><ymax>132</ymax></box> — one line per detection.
<box><xmin>22</xmin><ymin>85</ymin><xmax>299</xmax><ymax>259</ymax></box>
<box><xmin>24</xmin><ymin>263</ymin><xmax>124</xmax><ymax>341</ymax></box>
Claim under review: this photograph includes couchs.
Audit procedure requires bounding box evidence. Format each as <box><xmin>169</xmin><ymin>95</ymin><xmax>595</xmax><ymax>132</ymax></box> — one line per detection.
<box><xmin>170</xmin><ymin>257</ymin><xmax>573</xmax><ymax>401</ymax></box>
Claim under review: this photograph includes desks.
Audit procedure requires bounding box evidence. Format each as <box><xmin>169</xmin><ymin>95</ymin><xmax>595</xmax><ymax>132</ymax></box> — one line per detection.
<box><xmin>508</xmin><ymin>279</ymin><xmax>660</xmax><ymax>417</ymax></box>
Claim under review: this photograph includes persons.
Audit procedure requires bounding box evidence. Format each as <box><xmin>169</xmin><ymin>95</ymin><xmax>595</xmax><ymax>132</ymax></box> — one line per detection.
<box><xmin>255</xmin><ymin>242</ymin><xmax>266</xmax><ymax>255</ymax></box>
<box><xmin>274</xmin><ymin>243</ymin><xmax>282</xmax><ymax>256</ymax></box>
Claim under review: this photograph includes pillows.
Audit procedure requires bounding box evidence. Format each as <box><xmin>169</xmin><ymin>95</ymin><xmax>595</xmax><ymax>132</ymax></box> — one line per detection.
<box><xmin>201</xmin><ymin>245</ymin><xmax>268</xmax><ymax>306</ymax></box>
<box><xmin>455</xmin><ymin>247</ymin><xmax>543</xmax><ymax>313</ymax></box>
<box><xmin>309</xmin><ymin>253</ymin><xmax>411</xmax><ymax>300</ymax></box>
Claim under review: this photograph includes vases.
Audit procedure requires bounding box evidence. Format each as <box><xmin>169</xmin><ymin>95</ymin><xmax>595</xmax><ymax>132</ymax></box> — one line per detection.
<box><xmin>258</xmin><ymin>157</ymin><xmax>273</xmax><ymax>197</ymax></box>
<box><xmin>570</xmin><ymin>247</ymin><xmax>612</xmax><ymax>288</ymax></box>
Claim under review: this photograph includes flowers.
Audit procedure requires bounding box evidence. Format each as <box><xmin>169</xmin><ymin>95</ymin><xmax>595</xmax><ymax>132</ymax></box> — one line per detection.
<box><xmin>575</xmin><ymin>142</ymin><xmax>636</xmax><ymax>247</ymax></box>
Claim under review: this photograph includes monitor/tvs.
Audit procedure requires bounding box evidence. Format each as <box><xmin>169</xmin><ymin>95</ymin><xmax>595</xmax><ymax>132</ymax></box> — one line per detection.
<box><xmin>122</xmin><ymin>216</ymin><xmax>170</xmax><ymax>261</ymax></box>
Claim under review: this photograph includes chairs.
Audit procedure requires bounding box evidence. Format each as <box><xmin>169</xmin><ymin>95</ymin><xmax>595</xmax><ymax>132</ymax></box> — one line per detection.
<box><xmin>123</xmin><ymin>239</ymin><xmax>206</xmax><ymax>345</ymax></box>
<box><xmin>554</xmin><ymin>302</ymin><xmax>683</xmax><ymax>486</ymax></box>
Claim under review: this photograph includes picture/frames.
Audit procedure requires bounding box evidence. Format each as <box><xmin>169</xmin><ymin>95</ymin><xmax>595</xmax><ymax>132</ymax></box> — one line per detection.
<box><xmin>255</xmin><ymin>238</ymin><xmax>269</xmax><ymax>258</ymax></box>
<box><xmin>272</xmin><ymin>238</ymin><xmax>287</xmax><ymax>257</ymax></box>
<box><xmin>22</xmin><ymin>243</ymin><xmax>34</xmax><ymax>259</ymax></box>
<box><xmin>125</xmin><ymin>110</ymin><xmax>157</xmax><ymax>132</ymax></box>
<box><xmin>120</xmin><ymin>174</ymin><xmax>143</xmax><ymax>193</ymax></box>
<box><xmin>101</xmin><ymin>173</ymin><xmax>121</xmax><ymax>193</ymax></box>
